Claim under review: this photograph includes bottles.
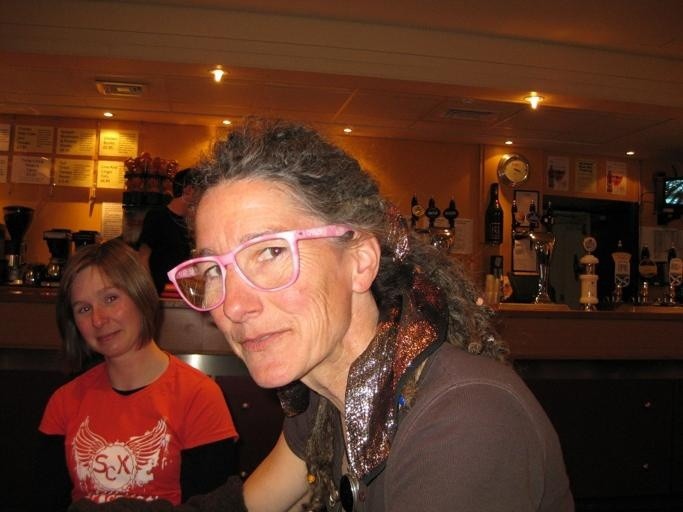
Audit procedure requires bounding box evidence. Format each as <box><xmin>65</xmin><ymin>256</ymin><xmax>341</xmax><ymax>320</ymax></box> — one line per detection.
<box><xmin>484</xmin><ymin>182</ymin><xmax>504</xmax><ymax>247</ymax></box>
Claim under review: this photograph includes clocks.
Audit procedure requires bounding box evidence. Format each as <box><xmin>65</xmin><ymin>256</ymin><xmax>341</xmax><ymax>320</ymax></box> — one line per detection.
<box><xmin>497</xmin><ymin>153</ymin><xmax>531</xmax><ymax>189</ymax></box>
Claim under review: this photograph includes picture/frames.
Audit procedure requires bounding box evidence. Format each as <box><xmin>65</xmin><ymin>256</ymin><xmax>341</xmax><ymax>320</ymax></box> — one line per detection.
<box><xmin>511</xmin><ymin>190</ymin><xmax>540</xmax><ymax>273</ymax></box>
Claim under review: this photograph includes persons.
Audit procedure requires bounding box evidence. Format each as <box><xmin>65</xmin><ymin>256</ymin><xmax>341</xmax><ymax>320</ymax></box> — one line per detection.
<box><xmin>138</xmin><ymin>164</ymin><xmax>207</xmax><ymax>296</ymax></box>
<box><xmin>164</xmin><ymin>116</ymin><xmax>575</xmax><ymax>509</ymax></box>
<box><xmin>34</xmin><ymin>236</ymin><xmax>241</xmax><ymax>509</ymax></box>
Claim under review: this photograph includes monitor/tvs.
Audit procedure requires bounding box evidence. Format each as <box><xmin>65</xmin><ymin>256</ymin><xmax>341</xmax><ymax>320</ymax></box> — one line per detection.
<box><xmin>663</xmin><ymin>177</ymin><xmax>683</xmax><ymax>209</ymax></box>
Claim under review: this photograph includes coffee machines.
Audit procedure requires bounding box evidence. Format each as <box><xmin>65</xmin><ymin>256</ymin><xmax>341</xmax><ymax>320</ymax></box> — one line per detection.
<box><xmin>38</xmin><ymin>228</ymin><xmax>74</xmax><ymax>287</ymax></box>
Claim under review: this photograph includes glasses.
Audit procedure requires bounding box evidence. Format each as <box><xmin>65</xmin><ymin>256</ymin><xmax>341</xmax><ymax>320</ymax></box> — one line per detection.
<box><xmin>166</xmin><ymin>223</ymin><xmax>353</xmax><ymax>313</ymax></box>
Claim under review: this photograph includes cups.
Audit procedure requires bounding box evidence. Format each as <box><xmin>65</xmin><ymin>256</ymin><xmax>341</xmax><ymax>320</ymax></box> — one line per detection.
<box><xmin>484</xmin><ymin>274</ymin><xmax>501</xmax><ymax>305</ymax></box>
